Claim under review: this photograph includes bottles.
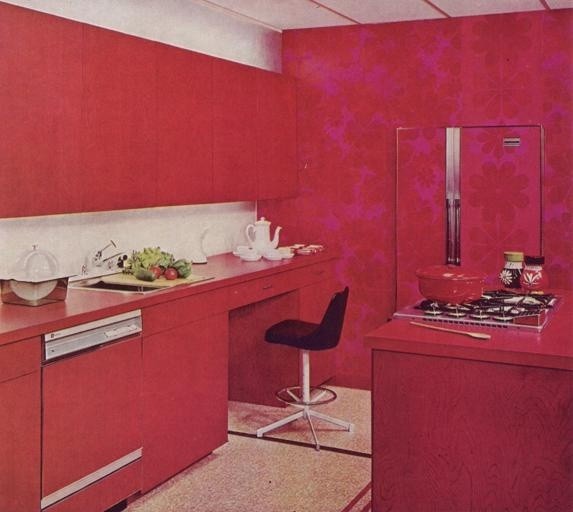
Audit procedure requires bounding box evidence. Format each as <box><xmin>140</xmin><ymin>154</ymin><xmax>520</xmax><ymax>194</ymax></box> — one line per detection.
<box><xmin>517</xmin><ymin>254</ymin><xmax>552</xmax><ymax>295</ymax></box>
<box><xmin>500</xmin><ymin>250</ymin><xmax>524</xmax><ymax>291</ymax></box>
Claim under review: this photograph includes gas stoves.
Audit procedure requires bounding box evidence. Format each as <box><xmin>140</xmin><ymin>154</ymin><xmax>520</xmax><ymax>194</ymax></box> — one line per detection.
<box><xmin>388</xmin><ymin>291</ymin><xmax>566</xmax><ymax>333</ymax></box>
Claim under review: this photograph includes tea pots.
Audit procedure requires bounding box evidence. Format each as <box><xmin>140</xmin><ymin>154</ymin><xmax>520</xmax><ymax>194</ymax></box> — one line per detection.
<box><xmin>244</xmin><ymin>215</ymin><xmax>283</xmax><ymax>250</ymax></box>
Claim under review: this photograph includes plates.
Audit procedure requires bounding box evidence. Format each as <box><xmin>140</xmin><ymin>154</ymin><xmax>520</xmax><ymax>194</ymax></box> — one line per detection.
<box><xmin>233</xmin><ymin>247</ymin><xmax>295</xmax><ymax>262</ymax></box>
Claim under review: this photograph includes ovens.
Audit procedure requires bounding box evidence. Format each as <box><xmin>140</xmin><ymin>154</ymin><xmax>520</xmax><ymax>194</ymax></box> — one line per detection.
<box><xmin>33</xmin><ymin>308</ymin><xmax>144</xmax><ymax>512</ymax></box>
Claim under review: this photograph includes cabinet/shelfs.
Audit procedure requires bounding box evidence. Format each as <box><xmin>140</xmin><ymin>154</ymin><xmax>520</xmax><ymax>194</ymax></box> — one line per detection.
<box><xmin>82</xmin><ymin>20</ymin><xmax>212</xmax><ymax>212</ymax></box>
<box><xmin>212</xmin><ymin>54</ymin><xmax>295</xmax><ymax>205</ymax></box>
<box><xmin>0</xmin><ymin>3</ymin><xmax>82</xmax><ymax>219</ymax></box>
<box><xmin>142</xmin><ymin>281</ymin><xmax>233</xmax><ymax>496</ymax></box>
<box><xmin>227</xmin><ymin>258</ymin><xmax>332</xmax><ymax>410</ymax></box>
<box><xmin>1</xmin><ymin>327</ymin><xmax>42</xmax><ymax>512</ymax></box>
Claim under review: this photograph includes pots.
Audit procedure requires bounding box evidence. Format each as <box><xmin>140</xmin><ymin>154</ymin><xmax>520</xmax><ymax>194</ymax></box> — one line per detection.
<box><xmin>415</xmin><ymin>262</ymin><xmax>489</xmax><ymax>304</ymax></box>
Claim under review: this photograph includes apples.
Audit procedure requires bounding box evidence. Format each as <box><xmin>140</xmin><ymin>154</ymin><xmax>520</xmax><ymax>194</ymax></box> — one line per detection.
<box><xmin>165</xmin><ymin>268</ymin><xmax>177</xmax><ymax>280</ymax></box>
<box><xmin>149</xmin><ymin>267</ymin><xmax>160</xmax><ymax>278</ymax></box>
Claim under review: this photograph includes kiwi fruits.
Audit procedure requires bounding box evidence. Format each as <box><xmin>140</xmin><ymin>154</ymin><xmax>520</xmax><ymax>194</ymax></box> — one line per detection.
<box><xmin>134</xmin><ymin>271</ymin><xmax>154</xmax><ymax>282</ymax></box>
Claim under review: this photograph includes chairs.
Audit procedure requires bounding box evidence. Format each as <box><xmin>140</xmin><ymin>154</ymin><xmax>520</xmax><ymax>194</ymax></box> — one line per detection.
<box><xmin>254</xmin><ymin>284</ymin><xmax>359</xmax><ymax>453</ymax></box>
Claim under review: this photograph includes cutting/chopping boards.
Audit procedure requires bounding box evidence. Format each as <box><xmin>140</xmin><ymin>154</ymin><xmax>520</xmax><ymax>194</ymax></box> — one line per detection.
<box><xmin>101</xmin><ymin>270</ymin><xmax>217</xmax><ymax>290</ymax></box>
